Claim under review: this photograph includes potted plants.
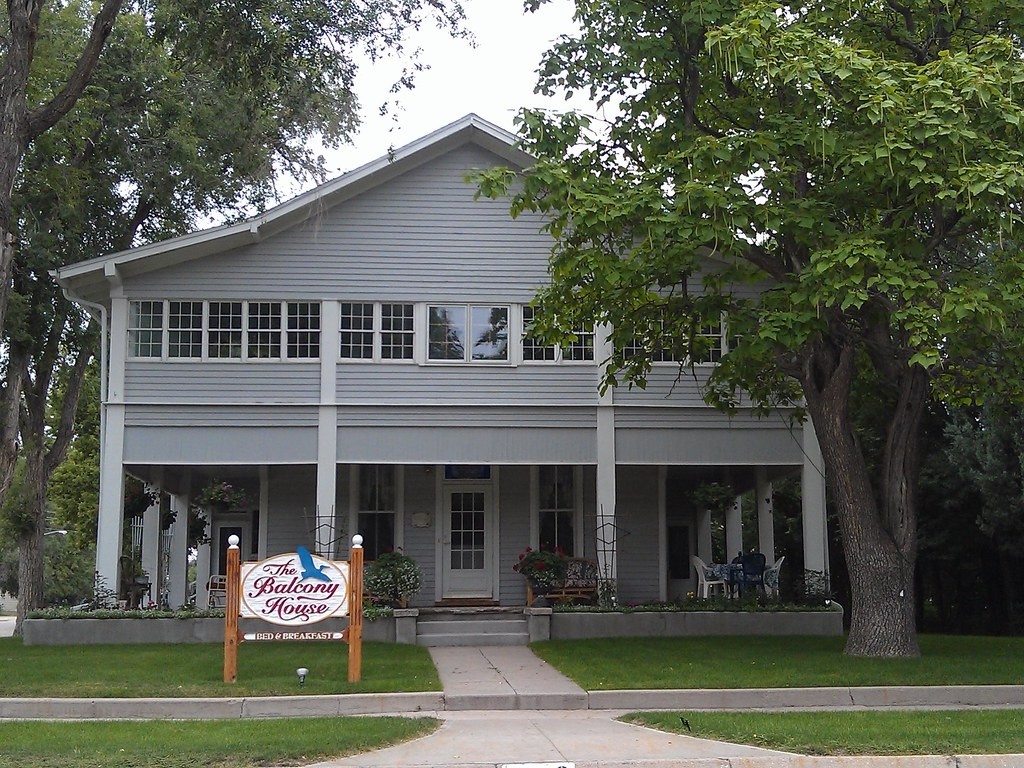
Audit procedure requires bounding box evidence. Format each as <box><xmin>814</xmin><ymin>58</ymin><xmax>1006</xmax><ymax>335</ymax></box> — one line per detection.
<box><xmin>135</xmin><ymin>565</ymin><xmax>150</xmax><ymax>584</ymax></box>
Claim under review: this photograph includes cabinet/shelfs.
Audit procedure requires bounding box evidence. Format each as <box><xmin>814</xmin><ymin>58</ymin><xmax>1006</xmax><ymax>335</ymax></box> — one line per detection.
<box><xmin>526</xmin><ymin>583</ymin><xmax>596</xmax><ymax>605</ymax></box>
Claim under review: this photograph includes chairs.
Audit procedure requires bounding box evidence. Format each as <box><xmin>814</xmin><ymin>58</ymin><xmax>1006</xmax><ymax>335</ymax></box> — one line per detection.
<box><xmin>730</xmin><ymin>552</ymin><xmax>766</xmax><ymax>595</ymax></box>
<box><xmin>764</xmin><ymin>555</ymin><xmax>785</xmax><ymax>596</ymax></box>
<box><xmin>690</xmin><ymin>554</ymin><xmax>724</xmax><ymax>599</ymax></box>
<box><xmin>206</xmin><ymin>575</ymin><xmax>226</xmax><ymax>609</ymax></box>
<box><xmin>731</xmin><ymin>552</ymin><xmax>743</xmax><ymax>565</ymax></box>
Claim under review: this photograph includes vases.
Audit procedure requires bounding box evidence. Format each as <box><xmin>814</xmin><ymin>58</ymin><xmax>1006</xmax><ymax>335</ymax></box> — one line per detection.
<box><xmin>530</xmin><ymin>595</ymin><xmax>551</xmax><ymax>608</ymax></box>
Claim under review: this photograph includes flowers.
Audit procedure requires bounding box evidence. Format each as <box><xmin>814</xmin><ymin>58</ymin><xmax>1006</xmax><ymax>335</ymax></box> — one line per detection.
<box><xmin>512</xmin><ymin>546</ymin><xmax>569</xmax><ymax>595</ymax></box>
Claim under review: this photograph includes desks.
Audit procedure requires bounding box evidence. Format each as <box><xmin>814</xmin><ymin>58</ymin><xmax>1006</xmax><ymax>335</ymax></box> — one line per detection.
<box><xmin>706</xmin><ymin>563</ymin><xmax>778</xmax><ymax>590</ymax></box>
